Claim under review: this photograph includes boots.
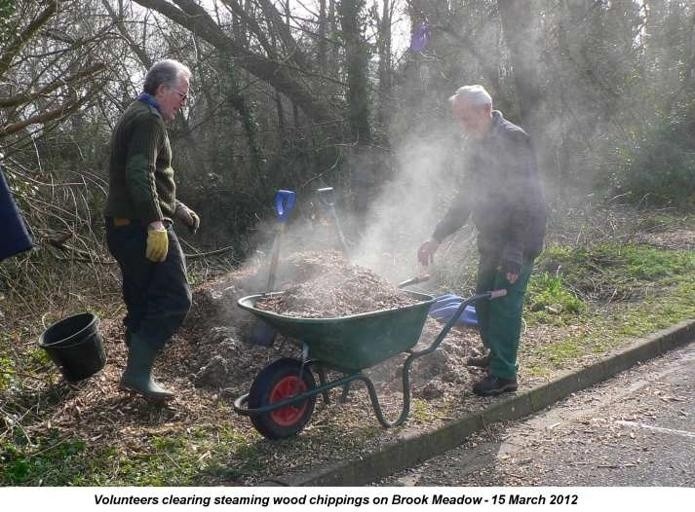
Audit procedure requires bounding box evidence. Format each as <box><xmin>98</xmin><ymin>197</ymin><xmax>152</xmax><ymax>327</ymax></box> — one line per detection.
<box><xmin>119</xmin><ymin>331</ymin><xmax>175</xmax><ymax>399</ymax></box>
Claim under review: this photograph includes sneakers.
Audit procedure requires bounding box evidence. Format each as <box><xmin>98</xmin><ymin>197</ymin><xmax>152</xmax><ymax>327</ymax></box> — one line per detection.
<box><xmin>465</xmin><ymin>351</ymin><xmax>492</xmax><ymax>367</ymax></box>
<box><xmin>473</xmin><ymin>376</ymin><xmax>518</xmax><ymax>395</ymax></box>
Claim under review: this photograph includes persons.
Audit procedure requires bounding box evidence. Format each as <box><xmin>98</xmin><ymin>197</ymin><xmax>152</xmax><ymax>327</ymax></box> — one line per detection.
<box><xmin>416</xmin><ymin>83</ymin><xmax>550</xmax><ymax>397</ymax></box>
<box><xmin>101</xmin><ymin>60</ymin><xmax>203</xmax><ymax>402</ymax></box>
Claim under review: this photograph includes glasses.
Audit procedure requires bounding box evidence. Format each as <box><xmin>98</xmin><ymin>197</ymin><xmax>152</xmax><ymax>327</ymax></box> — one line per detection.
<box><xmin>173</xmin><ymin>90</ymin><xmax>188</xmax><ymax>102</ymax></box>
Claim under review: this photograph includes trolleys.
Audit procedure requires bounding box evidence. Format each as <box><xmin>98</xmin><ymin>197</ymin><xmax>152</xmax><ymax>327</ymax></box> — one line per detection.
<box><xmin>230</xmin><ymin>270</ymin><xmax>511</xmax><ymax>438</ymax></box>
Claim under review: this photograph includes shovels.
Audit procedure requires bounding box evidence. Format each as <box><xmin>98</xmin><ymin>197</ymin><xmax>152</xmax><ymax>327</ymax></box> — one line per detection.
<box><xmin>243</xmin><ymin>189</ymin><xmax>295</xmax><ymax>345</ymax></box>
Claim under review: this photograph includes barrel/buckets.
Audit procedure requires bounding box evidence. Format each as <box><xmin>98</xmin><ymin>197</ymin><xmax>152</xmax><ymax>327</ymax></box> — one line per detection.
<box><xmin>38</xmin><ymin>312</ymin><xmax>106</xmax><ymax>380</ymax></box>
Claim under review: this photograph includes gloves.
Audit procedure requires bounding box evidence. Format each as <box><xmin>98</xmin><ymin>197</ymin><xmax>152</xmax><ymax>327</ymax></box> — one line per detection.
<box><xmin>499</xmin><ymin>242</ymin><xmax>525</xmax><ymax>272</ymax></box>
<box><xmin>146</xmin><ymin>229</ymin><xmax>169</xmax><ymax>262</ymax></box>
<box><xmin>176</xmin><ymin>205</ymin><xmax>200</xmax><ymax>234</ymax></box>
